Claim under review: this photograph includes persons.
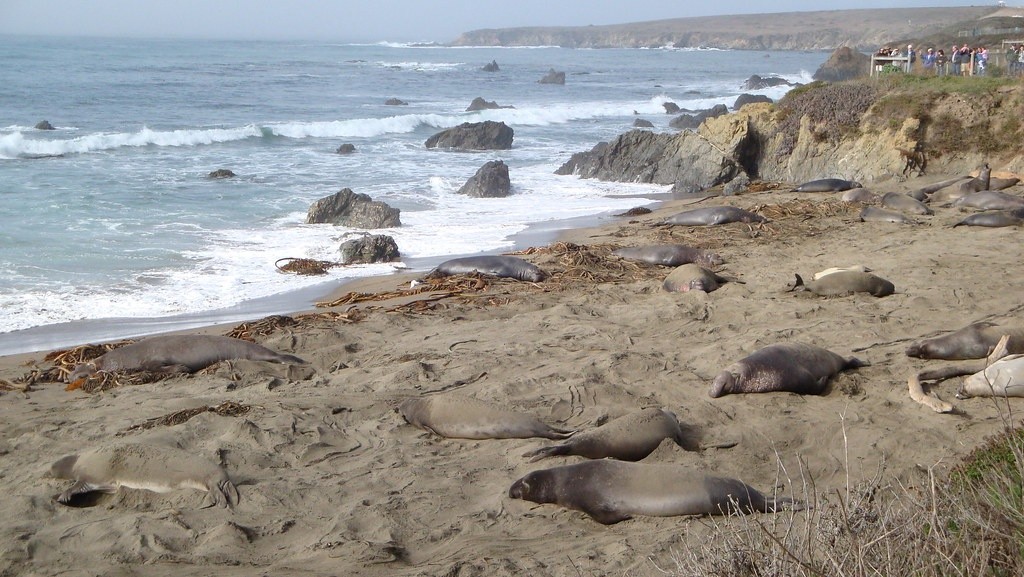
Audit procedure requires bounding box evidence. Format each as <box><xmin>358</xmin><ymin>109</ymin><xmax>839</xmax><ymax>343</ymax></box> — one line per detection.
<box><xmin>1006</xmin><ymin>43</ymin><xmax>1020</xmax><ymax>77</ymax></box>
<box><xmin>904</xmin><ymin>46</ymin><xmax>917</xmax><ymax>75</ymax></box>
<box><xmin>970</xmin><ymin>48</ymin><xmax>977</xmax><ymax>75</ymax></box>
<box><xmin>875</xmin><ymin>47</ymin><xmax>895</xmax><ymax>76</ymax></box>
<box><xmin>919</xmin><ymin>48</ymin><xmax>936</xmax><ymax>76</ymax></box>
<box><xmin>979</xmin><ymin>47</ymin><xmax>989</xmax><ymax>71</ymax></box>
<box><xmin>892</xmin><ymin>48</ymin><xmax>903</xmax><ymax>70</ymax></box>
<box><xmin>959</xmin><ymin>44</ymin><xmax>973</xmax><ymax>77</ymax></box>
<box><xmin>1015</xmin><ymin>45</ymin><xmax>1024</xmax><ymax>76</ymax></box>
<box><xmin>948</xmin><ymin>46</ymin><xmax>962</xmax><ymax>77</ymax></box>
<box><xmin>975</xmin><ymin>47</ymin><xmax>987</xmax><ymax>77</ymax></box>
<box><xmin>935</xmin><ymin>50</ymin><xmax>949</xmax><ymax>78</ymax></box>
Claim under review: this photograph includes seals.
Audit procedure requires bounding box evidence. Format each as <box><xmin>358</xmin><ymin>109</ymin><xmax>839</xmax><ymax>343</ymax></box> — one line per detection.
<box><xmin>50</xmin><ymin>162</ymin><xmax>1024</xmax><ymax>518</ymax></box>
